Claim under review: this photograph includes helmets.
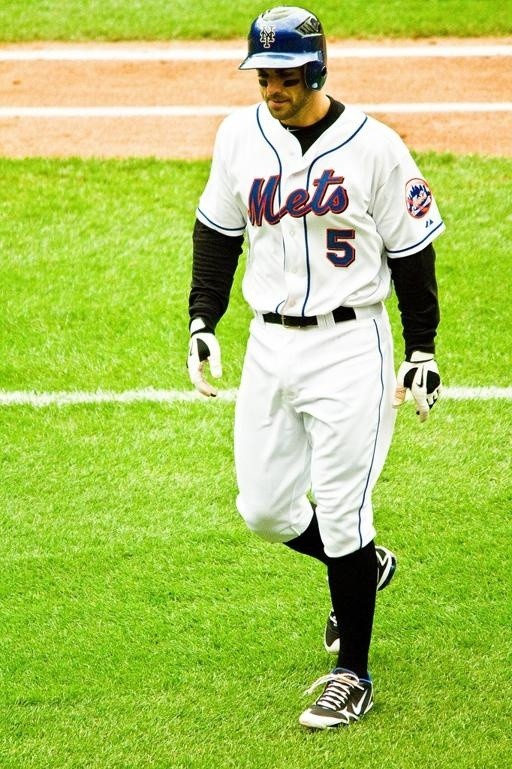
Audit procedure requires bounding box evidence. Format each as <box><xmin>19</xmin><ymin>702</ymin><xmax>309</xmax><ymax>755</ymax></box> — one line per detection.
<box><xmin>238</xmin><ymin>6</ymin><xmax>327</xmax><ymax>91</ymax></box>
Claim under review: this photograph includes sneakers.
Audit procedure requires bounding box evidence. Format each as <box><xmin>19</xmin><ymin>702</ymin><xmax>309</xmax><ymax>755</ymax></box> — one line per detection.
<box><xmin>324</xmin><ymin>545</ymin><xmax>396</xmax><ymax>655</ymax></box>
<box><xmin>298</xmin><ymin>667</ymin><xmax>374</xmax><ymax>730</ymax></box>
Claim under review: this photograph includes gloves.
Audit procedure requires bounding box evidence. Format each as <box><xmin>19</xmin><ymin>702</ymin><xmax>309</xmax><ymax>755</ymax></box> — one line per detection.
<box><xmin>186</xmin><ymin>316</ymin><xmax>222</xmax><ymax>397</ymax></box>
<box><xmin>392</xmin><ymin>350</ymin><xmax>440</xmax><ymax>423</ymax></box>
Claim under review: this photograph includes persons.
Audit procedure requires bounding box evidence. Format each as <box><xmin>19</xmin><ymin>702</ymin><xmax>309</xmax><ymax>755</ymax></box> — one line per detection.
<box><xmin>186</xmin><ymin>6</ymin><xmax>443</xmax><ymax>735</ymax></box>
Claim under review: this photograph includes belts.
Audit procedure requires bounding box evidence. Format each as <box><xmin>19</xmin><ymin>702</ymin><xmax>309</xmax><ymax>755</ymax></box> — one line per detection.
<box><xmin>263</xmin><ymin>306</ymin><xmax>356</xmax><ymax>329</ymax></box>
